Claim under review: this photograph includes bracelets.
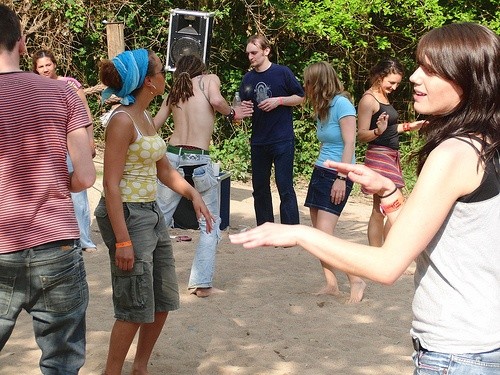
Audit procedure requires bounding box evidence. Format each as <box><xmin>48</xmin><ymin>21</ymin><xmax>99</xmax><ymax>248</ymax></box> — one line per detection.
<box><xmin>374</xmin><ymin>128</ymin><xmax>381</xmax><ymax>137</ymax></box>
<box><xmin>403</xmin><ymin>123</ymin><xmax>411</xmax><ymax>132</ymax></box>
<box><xmin>279</xmin><ymin>96</ymin><xmax>286</xmax><ymax>105</ymax></box>
<box><xmin>335</xmin><ymin>174</ymin><xmax>348</xmax><ymax>182</ymax></box>
<box><xmin>380</xmin><ymin>194</ymin><xmax>405</xmax><ymax>217</ymax></box>
<box><xmin>226</xmin><ymin>108</ymin><xmax>235</xmax><ymax>120</ymax></box>
<box><xmin>115</xmin><ymin>240</ymin><xmax>132</xmax><ymax>248</ymax></box>
<box><xmin>378</xmin><ymin>187</ymin><xmax>397</xmax><ymax>198</ymax></box>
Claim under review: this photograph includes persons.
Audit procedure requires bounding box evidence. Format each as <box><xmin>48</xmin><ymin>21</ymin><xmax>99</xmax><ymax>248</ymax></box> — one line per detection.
<box><xmin>227</xmin><ymin>22</ymin><xmax>500</xmax><ymax>375</ymax></box>
<box><xmin>32</xmin><ymin>50</ymin><xmax>98</xmax><ymax>253</ymax></box>
<box><xmin>237</xmin><ymin>34</ymin><xmax>307</xmax><ymax>249</ymax></box>
<box><xmin>152</xmin><ymin>54</ymin><xmax>254</xmax><ymax>297</ymax></box>
<box><xmin>1</xmin><ymin>3</ymin><xmax>95</xmax><ymax>375</ymax></box>
<box><xmin>357</xmin><ymin>59</ymin><xmax>428</xmax><ymax>277</ymax></box>
<box><xmin>301</xmin><ymin>61</ymin><xmax>366</xmax><ymax>304</ymax></box>
<box><xmin>93</xmin><ymin>48</ymin><xmax>216</xmax><ymax>375</ymax></box>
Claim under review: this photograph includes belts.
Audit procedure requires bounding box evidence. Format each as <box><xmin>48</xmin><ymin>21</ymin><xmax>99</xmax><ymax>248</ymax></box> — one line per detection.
<box><xmin>166</xmin><ymin>145</ymin><xmax>211</xmax><ymax>156</ymax></box>
<box><xmin>33</xmin><ymin>238</ymin><xmax>75</xmax><ymax>251</ymax></box>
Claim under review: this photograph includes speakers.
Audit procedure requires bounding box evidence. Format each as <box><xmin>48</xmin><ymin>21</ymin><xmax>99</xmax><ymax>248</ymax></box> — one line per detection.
<box><xmin>164</xmin><ymin>9</ymin><xmax>216</xmax><ymax>74</ymax></box>
